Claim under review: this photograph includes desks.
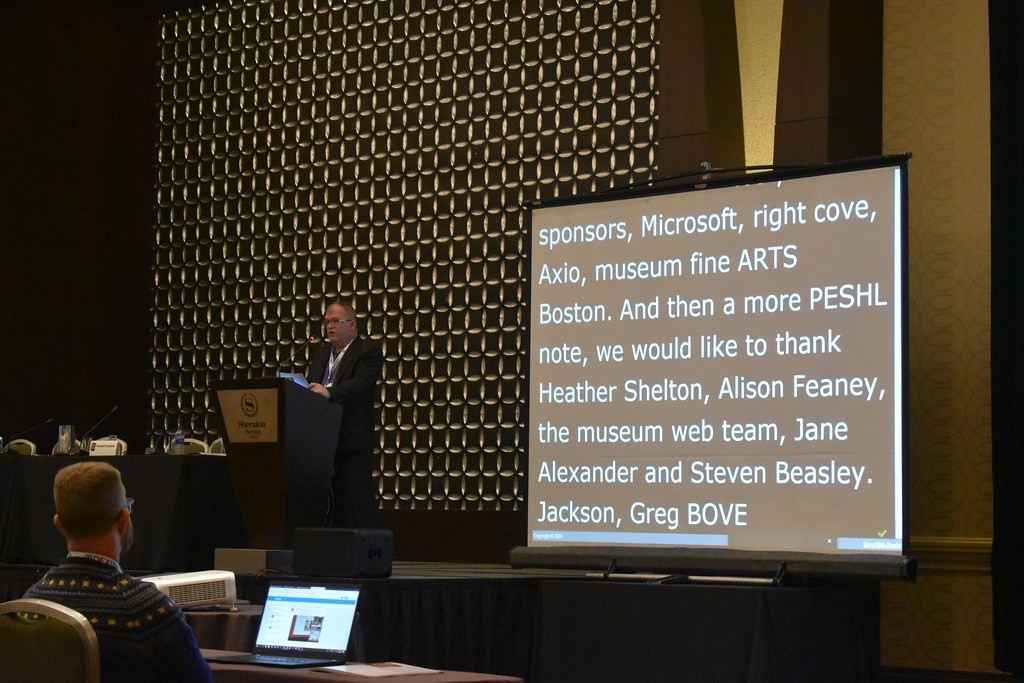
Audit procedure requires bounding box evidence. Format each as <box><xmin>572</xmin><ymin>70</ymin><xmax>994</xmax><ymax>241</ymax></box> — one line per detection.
<box><xmin>229</xmin><ymin>599</ymin><xmax>251</xmax><ymax>604</ymax></box>
<box><xmin>183</xmin><ymin>605</ymin><xmax>363</xmax><ymax>662</ymax></box>
<box><xmin>0</xmin><ymin>456</ymin><xmax>247</xmax><ymax>570</ymax></box>
<box><xmin>200</xmin><ymin>647</ymin><xmax>523</xmax><ymax>683</ymax></box>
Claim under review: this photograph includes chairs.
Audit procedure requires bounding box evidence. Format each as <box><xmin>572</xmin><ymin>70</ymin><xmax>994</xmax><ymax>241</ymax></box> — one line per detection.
<box><xmin>0</xmin><ymin>597</ymin><xmax>100</xmax><ymax>683</ymax></box>
<box><xmin>3</xmin><ymin>438</ymin><xmax>36</xmax><ymax>455</ymax></box>
<box><xmin>209</xmin><ymin>437</ymin><xmax>226</xmax><ymax>454</ymax></box>
<box><xmin>91</xmin><ymin>436</ymin><xmax>128</xmax><ymax>456</ymax></box>
<box><xmin>52</xmin><ymin>439</ymin><xmax>82</xmax><ymax>454</ymax></box>
<box><xmin>166</xmin><ymin>438</ymin><xmax>209</xmax><ymax>454</ymax></box>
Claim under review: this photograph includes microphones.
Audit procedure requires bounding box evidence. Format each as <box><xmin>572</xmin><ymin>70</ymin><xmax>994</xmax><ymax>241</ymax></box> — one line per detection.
<box><xmin>272</xmin><ymin>334</ymin><xmax>317</xmax><ymax>378</ymax></box>
<box><xmin>83</xmin><ymin>406</ymin><xmax>119</xmax><ymax>439</ymax></box>
<box><xmin>10</xmin><ymin>418</ymin><xmax>54</xmax><ymax>442</ymax></box>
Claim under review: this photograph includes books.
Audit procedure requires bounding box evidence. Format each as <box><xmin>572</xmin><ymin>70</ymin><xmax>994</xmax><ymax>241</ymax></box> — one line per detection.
<box><xmin>308</xmin><ymin>660</ymin><xmax>445</xmax><ymax>678</ymax></box>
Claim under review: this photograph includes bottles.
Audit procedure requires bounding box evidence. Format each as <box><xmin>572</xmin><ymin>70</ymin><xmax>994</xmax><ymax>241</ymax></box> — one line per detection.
<box><xmin>175</xmin><ymin>426</ymin><xmax>184</xmax><ymax>455</ymax></box>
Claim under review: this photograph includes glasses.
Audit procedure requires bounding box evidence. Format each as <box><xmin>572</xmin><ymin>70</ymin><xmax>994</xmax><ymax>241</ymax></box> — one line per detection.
<box><xmin>121</xmin><ymin>498</ymin><xmax>134</xmax><ymax>511</ymax></box>
<box><xmin>324</xmin><ymin>319</ymin><xmax>353</xmax><ymax>326</ymax></box>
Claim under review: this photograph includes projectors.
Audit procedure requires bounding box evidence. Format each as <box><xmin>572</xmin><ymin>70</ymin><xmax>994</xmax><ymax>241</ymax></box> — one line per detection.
<box><xmin>140</xmin><ymin>570</ymin><xmax>236</xmax><ymax>609</ymax></box>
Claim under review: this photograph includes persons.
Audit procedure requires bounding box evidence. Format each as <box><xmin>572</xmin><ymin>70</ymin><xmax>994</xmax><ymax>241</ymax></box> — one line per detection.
<box><xmin>305</xmin><ymin>299</ymin><xmax>385</xmax><ymax>531</ymax></box>
<box><xmin>14</xmin><ymin>458</ymin><xmax>214</xmax><ymax>683</ymax></box>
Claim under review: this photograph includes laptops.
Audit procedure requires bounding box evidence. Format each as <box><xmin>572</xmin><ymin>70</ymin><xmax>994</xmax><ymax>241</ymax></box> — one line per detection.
<box><xmin>204</xmin><ymin>583</ymin><xmax>365</xmax><ymax>668</ymax></box>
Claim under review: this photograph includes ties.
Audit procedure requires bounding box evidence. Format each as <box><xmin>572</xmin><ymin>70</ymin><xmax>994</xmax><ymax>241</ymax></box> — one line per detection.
<box><xmin>333</xmin><ymin>350</ymin><xmax>341</xmax><ymax>363</ymax></box>
